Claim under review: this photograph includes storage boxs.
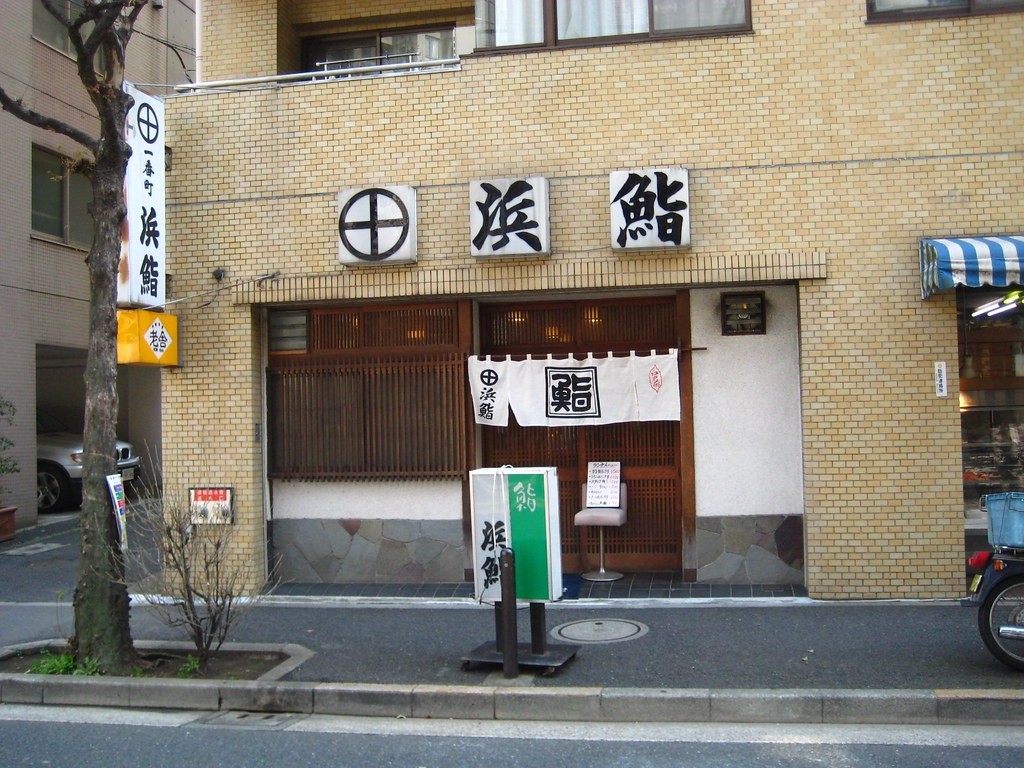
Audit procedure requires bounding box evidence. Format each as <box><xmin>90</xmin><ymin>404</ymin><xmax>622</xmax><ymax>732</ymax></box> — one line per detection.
<box><xmin>979</xmin><ymin>492</ymin><xmax>1024</xmax><ymax>548</ymax></box>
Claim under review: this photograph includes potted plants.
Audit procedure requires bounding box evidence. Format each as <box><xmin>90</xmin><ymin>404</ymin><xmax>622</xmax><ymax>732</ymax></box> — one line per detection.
<box><xmin>0</xmin><ymin>394</ymin><xmax>22</xmax><ymax>543</ymax></box>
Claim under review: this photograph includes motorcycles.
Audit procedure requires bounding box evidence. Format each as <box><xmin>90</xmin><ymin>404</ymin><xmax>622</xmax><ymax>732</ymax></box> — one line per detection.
<box><xmin>952</xmin><ymin>544</ymin><xmax>1024</xmax><ymax>674</ymax></box>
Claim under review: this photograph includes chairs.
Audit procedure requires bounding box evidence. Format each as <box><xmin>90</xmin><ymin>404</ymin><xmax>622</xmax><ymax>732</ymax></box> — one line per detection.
<box><xmin>574</xmin><ymin>483</ymin><xmax>629</xmax><ymax>583</ymax></box>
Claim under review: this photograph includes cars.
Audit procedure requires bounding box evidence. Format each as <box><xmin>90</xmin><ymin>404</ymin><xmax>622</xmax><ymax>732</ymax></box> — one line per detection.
<box><xmin>36</xmin><ymin>407</ymin><xmax>141</xmax><ymax>515</ymax></box>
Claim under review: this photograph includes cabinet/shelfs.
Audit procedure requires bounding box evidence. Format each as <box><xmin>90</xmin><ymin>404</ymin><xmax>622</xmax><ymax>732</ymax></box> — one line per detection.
<box><xmin>961</xmin><ymin>405</ymin><xmax>1024</xmax><ymax>529</ymax></box>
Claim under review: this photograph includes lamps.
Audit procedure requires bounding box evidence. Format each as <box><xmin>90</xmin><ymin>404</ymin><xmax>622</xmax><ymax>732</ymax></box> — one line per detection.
<box><xmin>959</xmin><ymin>287</ymin><xmax>976</xmax><ymax>378</ymax></box>
